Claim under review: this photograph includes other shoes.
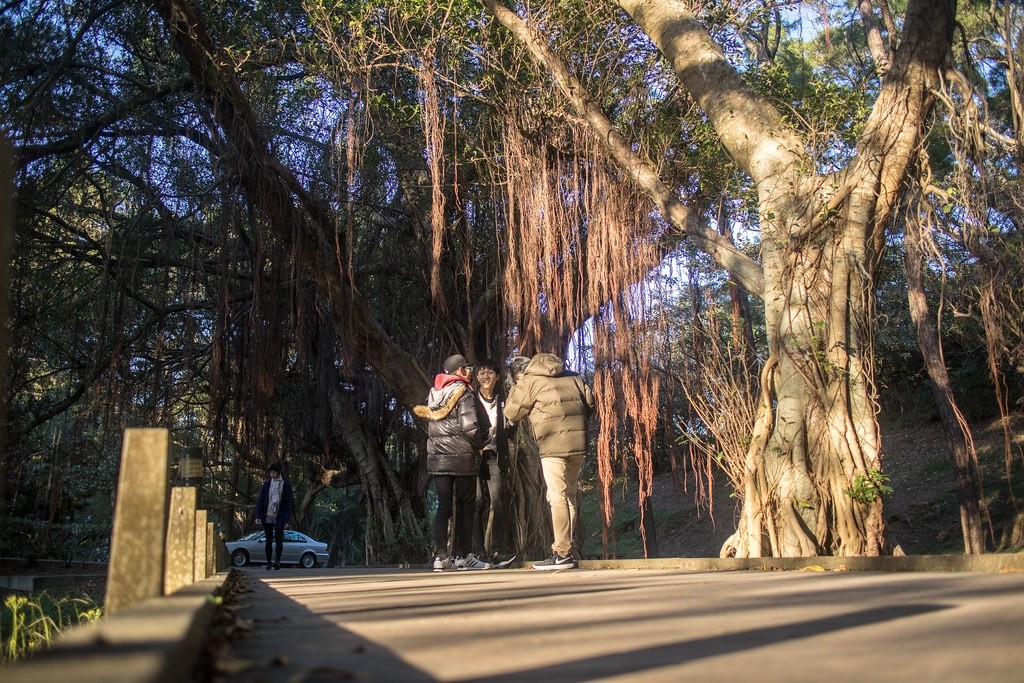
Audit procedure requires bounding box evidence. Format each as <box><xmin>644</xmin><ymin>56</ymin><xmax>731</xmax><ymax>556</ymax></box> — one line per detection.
<box><xmin>266</xmin><ymin>562</ymin><xmax>272</xmax><ymax>570</ymax></box>
<box><xmin>273</xmin><ymin>562</ymin><xmax>280</xmax><ymax>570</ymax></box>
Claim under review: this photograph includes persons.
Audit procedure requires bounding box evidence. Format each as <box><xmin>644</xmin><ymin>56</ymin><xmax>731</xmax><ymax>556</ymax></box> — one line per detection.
<box><xmin>504</xmin><ymin>353</ymin><xmax>596</xmax><ymax>570</ymax></box>
<box><xmin>426</xmin><ymin>354</ymin><xmax>490</xmax><ymax>572</ymax></box>
<box><xmin>256</xmin><ymin>463</ymin><xmax>292</xmax><ymax>571</ymax></box>
<box><xmin>471</xmin><ymin>358</ymin><xmax>516</xmax><ymax>567</ymax></box>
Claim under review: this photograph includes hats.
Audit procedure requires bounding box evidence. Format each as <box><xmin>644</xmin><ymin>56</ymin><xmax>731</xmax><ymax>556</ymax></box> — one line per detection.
<box><xmin>269</xmin><ymin>462</ymin><xmax>281</xmax><ymax>472</ymax></box>
<box><xmin>444</xmin><ymin>355</ymin><xmax>474</xmax><ymax>374</ymax></box>
<box><xmin>509</xmin><ymin>356</ymin><xmax>530</xmax><ymax>375</ymax></box>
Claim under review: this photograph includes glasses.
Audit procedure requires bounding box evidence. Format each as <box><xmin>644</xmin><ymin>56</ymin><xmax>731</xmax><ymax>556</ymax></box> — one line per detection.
<box><xmin>466</xmin><ymin>367</ymin><xmax>473</xmax><ymax>372</ymax></box>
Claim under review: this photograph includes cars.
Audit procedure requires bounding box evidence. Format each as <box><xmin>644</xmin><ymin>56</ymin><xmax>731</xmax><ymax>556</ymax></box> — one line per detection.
<box><xmin>226</xmin><ymin>528</ymin><xmax>330</xmax><ymax>569</ymax></box>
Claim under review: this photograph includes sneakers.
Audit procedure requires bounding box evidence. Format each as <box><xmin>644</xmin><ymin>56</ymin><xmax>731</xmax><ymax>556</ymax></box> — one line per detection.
<box><xmin>489</xmin><ymin>552</ymin><xmax>516</xmax><ymax>568</ymax></box>
<box><xmin>532</xmin><ymin>551</ymin><xmax>578</xmax><ymax>570</ymax></box>
<box><xmin>454</xmin><ymin>553</ymin><xmax>491</xmax><ymax>570</ymax></box>
<box><xmin>433</xmin><ymin>556</ymin><xmax>456</xmax><ymax>572</ymax></box>
<box><xmin>474</xmin><ymin>550</ymin><xmax>488</xmax><ymax>563</ymax></box>
